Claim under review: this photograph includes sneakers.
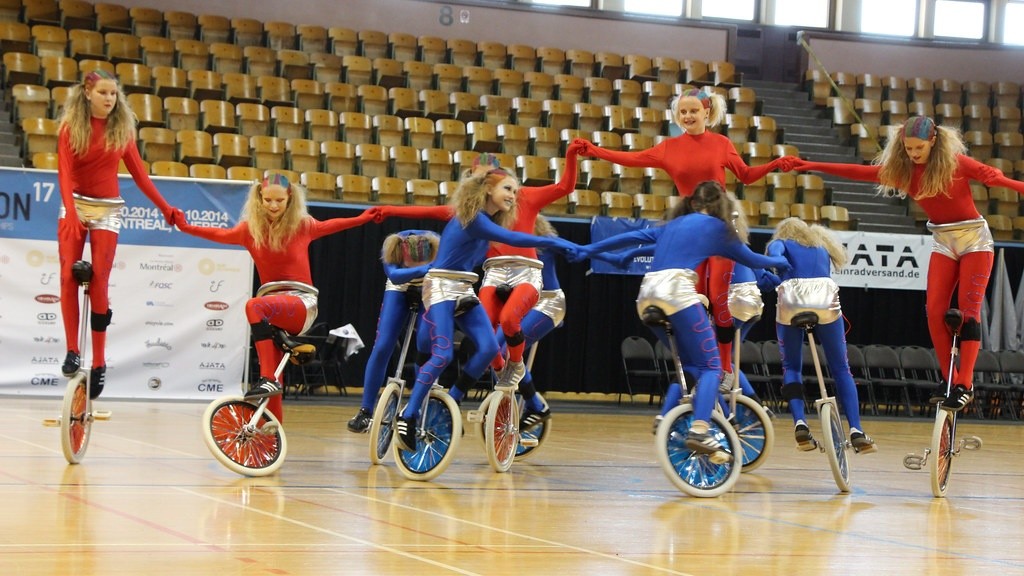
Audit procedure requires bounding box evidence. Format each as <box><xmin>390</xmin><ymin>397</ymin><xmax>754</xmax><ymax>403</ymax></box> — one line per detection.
<box><xmin>461</xmin><ymin>428</ymin><xmax>465</xmax><ymax>437</ymax></box>
<box><xmin>348</xmin><ymin>406</ymin><xmax>371</xmax><ymax>434</ymax></box>
<box><xmin>62</xmin><ymin>349</ymin><xmax>81</xmax><ymax>377</ymax></box>
<box><xmin>849</xmin><ymin>431</ymin><xmax>873</xmax><ymax>448</ymax></box>
<box><xmin>717</xmin><ymin>369</ymin><xmax>735</xmax><ymax>392</ymax></box>
<box><xmin>929</xmin><ymin>380</ymin><xmax>948</xmax><ymax>402</ymax></box>
<box><xmin>90</xmin><ymin>362</ymin><xmax>106</xmax><ymax>400</ymax></box>
<box><xmin>794</xmin><ymin>423</ymin><xmax>818</xmax><ymax>451</ymax></box>
<box><xmin>393</xmin><ymin>412</ymin><xmax>416</xmax><ymax>452</ymax></box>
<box><xmin>940</xmin><ymin>383</ymin><xmax>974</xmax><ymax>411</ymax></box>
<box><xmin>244</xmin><ymin>377</ymin><xmax>283</xmax><ymax>400</ymax></box>
<box><xmin>494</xmin><ymin>357</ymin><xmax>526</xmax><ymax>390</ymax></box>
<box><xmin>519</xmin><ymin>405</ymin><xmax>550</xmax><ymax>433</ymax></box>
<box><xmin>686</xmin><ymin>427</ymin><xmax>734</xmax><ymax>460</ymax></box>
<box><xmin>650</xmin><ymin>415</ymin><xmax>664</xmax><ymax>435</ymax></box>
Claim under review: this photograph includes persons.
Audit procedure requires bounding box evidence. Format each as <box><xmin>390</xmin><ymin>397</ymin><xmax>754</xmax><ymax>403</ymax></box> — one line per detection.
<box><xmin>172</xmin><ymin>172</ymin><xmax>381</xmax><ymax>425</ymax></box>
<box><xmin>346</xmin><ymin>137</ymin><xmax>587</xmax><ymax>451</ymax></box>
<box><xmin>58</xmin><ymin>66</ymin><xmax>178</xmax><ymax>399</ymax></box>
<box><xmin>793</xmin><ymin>116</ymin><xmax>1024</xmax><ymax>411</ymax></box>
<box><xmin>571</xmin><ymin>88</ymin><xmax>873</xmax><ymax>463</ymax></box>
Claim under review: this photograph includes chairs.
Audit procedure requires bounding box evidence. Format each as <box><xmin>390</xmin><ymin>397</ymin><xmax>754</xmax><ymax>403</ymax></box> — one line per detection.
<box><xmin>393</xmin><ymin>329</ymin><xmax>495</xmax><ymax>403</ymax></box>
<box><xmin>616</xmin><ymin>336</ymin><xmax>1024</xmax><ymax>420</ymax></box>
<box><xmin>248</xmin><ymin>321</ymin><xmax>365</xmax><ymax>401</ymax></box>
<box><xmin>0</xmin><ymin>0</ymin><xmax>1024</xmax><ymax>243</ymax></box>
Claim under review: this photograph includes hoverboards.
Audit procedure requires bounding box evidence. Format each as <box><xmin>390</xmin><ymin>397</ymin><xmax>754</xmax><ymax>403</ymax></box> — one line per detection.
<box><xmin>903</xmin><ymin>308</ymin><xmax>981</xmax><ymax>500</ymax></box>
<box><xmin>43</xmin><ymin>261</ymin><xmax>111</xmax><ymax>466</ymax></box>
<box><xmin>790</xmin><ymin>311</ymin><xmax>873</xmax><ymax>491</ymax></box>
<box><xmin>202</xmin><ymin>329</ymin><xmax>315</xmax><ymax>476</ymax></box>
<box><xmin>361</xmin><ymin>296</ymin><xmax>565</xmax><ymax>479</ymax></box>
<box><xmin>643</xmin><ymin>300</ymin><xmax>774</xmax><ymax>497</ymax></box>
